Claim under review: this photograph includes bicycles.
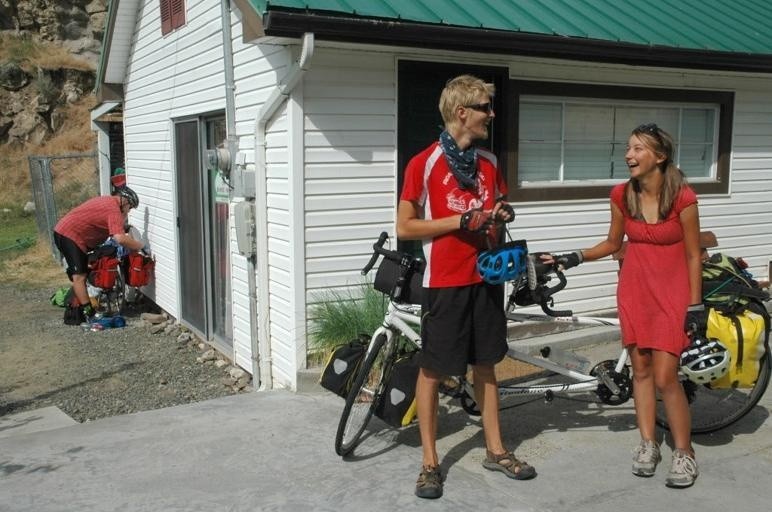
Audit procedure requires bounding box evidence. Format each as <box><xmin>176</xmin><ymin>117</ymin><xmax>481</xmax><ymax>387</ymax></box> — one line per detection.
<box><xmin>98</xmin><ymin>243</ymin><xmax>136</xmax><ymax>311</ymax></box>
<box><xmin>333</xmin><ymin>232</ymin><xmax>770</xmax><ymax>457</ymax></box>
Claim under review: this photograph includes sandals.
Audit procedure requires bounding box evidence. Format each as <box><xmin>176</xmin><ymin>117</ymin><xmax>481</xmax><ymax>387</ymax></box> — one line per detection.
<box><xmin>416</xmin><ymin>467</ymin><xmax>443</xmax><ymax>500</ymax></box>
<box><xmin>482</xmin><ymin>450</ymin><xmax>536</xmax><ymax>480</ymax></box>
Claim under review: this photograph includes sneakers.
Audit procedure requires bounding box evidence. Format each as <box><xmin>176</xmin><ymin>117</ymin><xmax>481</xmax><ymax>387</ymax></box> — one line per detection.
<box><xmin>666</xmin><ymin>450</ymin><xmax>699</xmax><ymax>488</ymax></box>
<box><xmin>632</xmin><ymin>441</ymin><xmax>662</xmax><ymax>477</ymax></box>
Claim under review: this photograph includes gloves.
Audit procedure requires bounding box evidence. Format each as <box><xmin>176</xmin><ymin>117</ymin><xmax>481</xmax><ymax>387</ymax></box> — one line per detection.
<box><xmin>460</xmin><ymin>208</ymin><xmax>494</xmax><ymax>234</ymax></box>
<box><xmin>495</xmin><ymin>200</ymin><xmax>515</xmax><ymax>222</ymax></box>
<box><xmin>551</xmin><ymin>249</ymin><xmax>583</xmax><ymax>272</ymax></box>
<box><xmin>685</xmin><ymin>304</ymin><xmax>707</xmax><ymax>338</ymax></box>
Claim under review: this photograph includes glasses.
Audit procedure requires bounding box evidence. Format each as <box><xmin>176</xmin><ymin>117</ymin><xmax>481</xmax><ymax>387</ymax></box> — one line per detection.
<box><xmin>464</xmin><ymin>102</ymin><xmax>494</xmax><ymax>112</ymax></box>
<box><xmin>638</xmin><ymin>122</ymin><xmax>666</xmax><ymax>148</ymax></box>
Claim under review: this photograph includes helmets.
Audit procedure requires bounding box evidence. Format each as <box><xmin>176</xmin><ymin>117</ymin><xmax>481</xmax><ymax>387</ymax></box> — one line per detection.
<box><xmin>476</xmin><ymin>240</ymin><xmax>528</xmax><ymax>285</ymax></box>
<box><xmin>680</xmin><ymin>339</ymin><xmax>731</xmax><ymax>384</ymax></box>
<box><xmin>118</xmin><ymin>186</ymin><xmax>138</xmax><ymax>208</ymax></box>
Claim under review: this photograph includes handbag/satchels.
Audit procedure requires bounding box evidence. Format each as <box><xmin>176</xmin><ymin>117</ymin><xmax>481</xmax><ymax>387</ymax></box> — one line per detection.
<box><xmin>706</xmin><ymin>308</ymin><xmax>768</xmax><ymax>389</ymax></box>
<box><xmin>374</xmin><ymin>349</ymin><xmax>421</xmax><ymax>429</ymax></box>
<box><xmin>127</xmin><ymin>251</ymin><xmax>155</xmax><ymax>287</ymax></box>
<box><xmin>320</xmin><ymin>333</ymin><xmax>372</xmax><ymax>400</ymax></box>
<box><xmin>91</xmin><ymin>256</ymin><xmax>118</xmax><ymax>289</ymax></box>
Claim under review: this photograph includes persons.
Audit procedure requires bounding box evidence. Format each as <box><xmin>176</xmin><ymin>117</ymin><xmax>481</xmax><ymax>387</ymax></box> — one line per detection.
<box><xmin>393</xmin><ymin>71</ymin><xmax>540</xmax><ymax>501</ymax></box>
<box><xmin>52</xmin><ymin>182</ymin><xmax>149</xmax><ymax>323</ymax></box>
<box><xmin>541</xmin><ymin>124</ymin><xmax>709</xmax><ymax>488</ymax></box>
